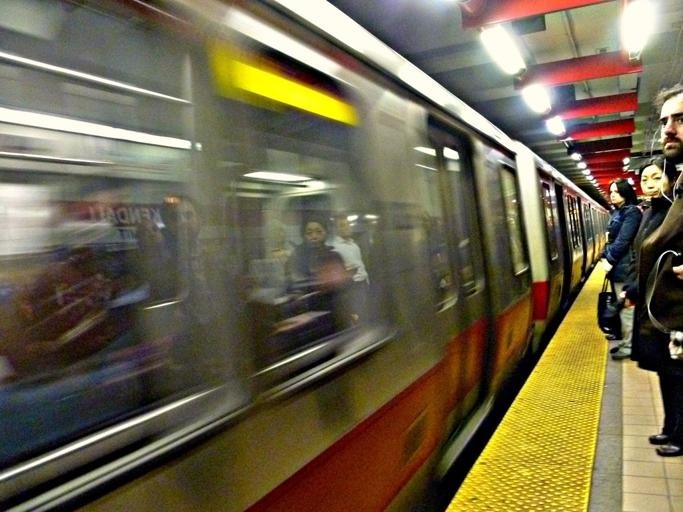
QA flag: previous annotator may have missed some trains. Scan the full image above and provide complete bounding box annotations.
[1,1,616,511]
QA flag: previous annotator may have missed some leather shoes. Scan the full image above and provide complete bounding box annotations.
[649,435,683,456]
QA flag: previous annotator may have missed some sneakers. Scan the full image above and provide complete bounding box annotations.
[610,347,628,360]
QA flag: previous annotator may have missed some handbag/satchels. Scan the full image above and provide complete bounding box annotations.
[597,276,622,340]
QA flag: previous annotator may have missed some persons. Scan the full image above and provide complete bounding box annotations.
[631,84,683,361]
[601,178,647,361]
[1,184,372,459]
[623,152,683,456]
